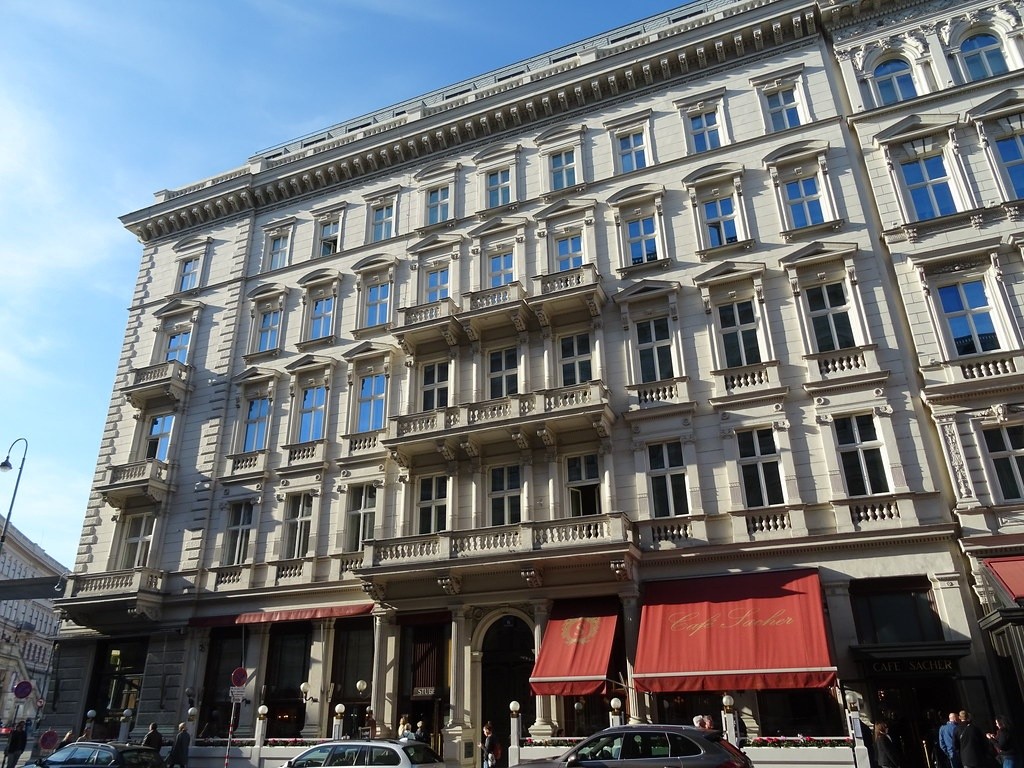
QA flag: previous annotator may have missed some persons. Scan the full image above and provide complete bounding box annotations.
[142,722,163,753]
[363,710,428,745]
[55,727,91,751]
[556,728,565,737]
[874,720,900,768]
[168,721,191,768]
[4,720,27,768]
[477,724,498,768]
[938,709,1020,768]
[692,714,714,731]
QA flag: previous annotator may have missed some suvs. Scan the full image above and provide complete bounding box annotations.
[277,739,446,768]
[18,741,166,768]
[509,724,755,768]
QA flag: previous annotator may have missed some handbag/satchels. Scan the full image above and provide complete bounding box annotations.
[487,753,496,767]
[995,754,1003,764]
[492,743,502,760]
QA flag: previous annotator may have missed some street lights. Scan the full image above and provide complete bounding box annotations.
[0,437,28,552]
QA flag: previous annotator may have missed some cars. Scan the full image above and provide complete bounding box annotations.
[0,719,18,736]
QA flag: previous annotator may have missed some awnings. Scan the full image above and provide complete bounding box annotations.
[633,567,839,692]
[234,602,373,624]
[527,600,621,696]
